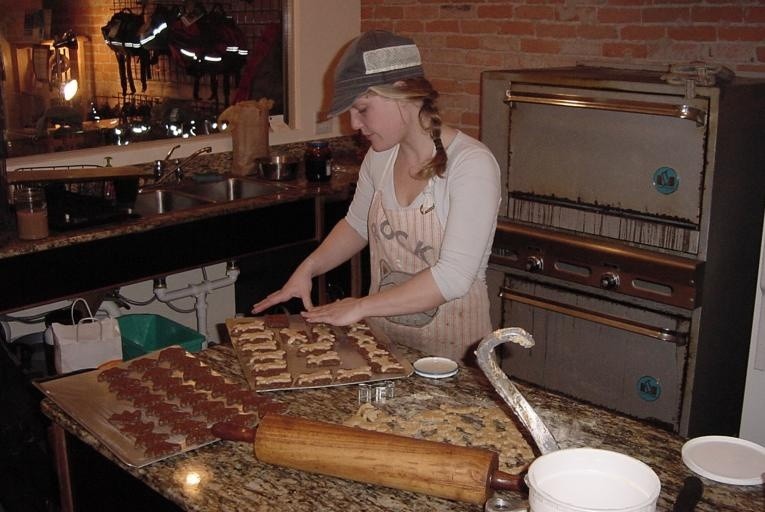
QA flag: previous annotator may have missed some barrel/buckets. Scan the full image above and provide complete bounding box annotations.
[522,447,661,512]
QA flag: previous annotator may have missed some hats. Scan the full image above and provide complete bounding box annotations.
[326,29,425,119]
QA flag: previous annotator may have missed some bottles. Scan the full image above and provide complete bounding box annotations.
[304,139,332,183]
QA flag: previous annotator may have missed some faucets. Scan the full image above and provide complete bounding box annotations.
[155,144,213,186]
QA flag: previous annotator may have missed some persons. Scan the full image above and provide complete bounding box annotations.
[251,30,501,364]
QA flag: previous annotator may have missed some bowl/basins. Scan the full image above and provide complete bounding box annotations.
[254,154,302,181]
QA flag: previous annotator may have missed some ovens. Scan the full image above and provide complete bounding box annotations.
[480,74,708,277]
[482,233,708,436]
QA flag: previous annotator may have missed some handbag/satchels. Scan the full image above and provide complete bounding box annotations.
[51,298,123,375]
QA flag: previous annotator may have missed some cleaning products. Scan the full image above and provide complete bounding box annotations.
[103,156,115,200]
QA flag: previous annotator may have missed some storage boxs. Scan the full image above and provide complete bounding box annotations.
[114,312,205,361]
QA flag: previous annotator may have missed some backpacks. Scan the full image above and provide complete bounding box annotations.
[101,0,246,74]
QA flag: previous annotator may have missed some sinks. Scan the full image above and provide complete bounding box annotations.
[133,186,216,218]
[176,173,297,203]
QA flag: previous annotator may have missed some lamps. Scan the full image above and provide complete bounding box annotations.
[50,35,78,102]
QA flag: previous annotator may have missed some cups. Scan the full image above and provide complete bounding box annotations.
[13,187,48,240]
